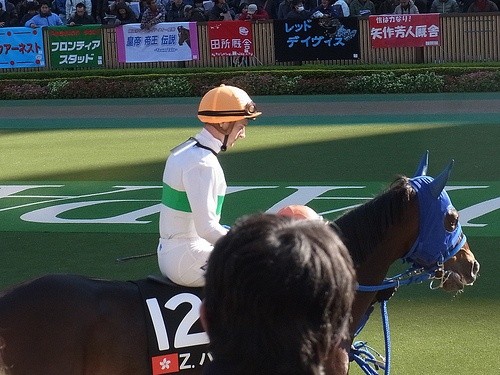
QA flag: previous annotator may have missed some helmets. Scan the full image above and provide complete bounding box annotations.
[197,83,262,125]
[276,205,323,220]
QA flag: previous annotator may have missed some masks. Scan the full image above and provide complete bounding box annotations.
[296,6,304,11]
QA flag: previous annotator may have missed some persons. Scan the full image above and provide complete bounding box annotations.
[157,84,262,287]
[200,213,358,375]
[0,0,500,30]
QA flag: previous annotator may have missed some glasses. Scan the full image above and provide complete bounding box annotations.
[244,100,258,115]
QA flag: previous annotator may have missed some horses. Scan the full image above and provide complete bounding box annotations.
[0,150,480,375]
[177,26,191,49]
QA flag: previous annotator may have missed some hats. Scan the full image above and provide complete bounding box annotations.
[248,4,257,14]
